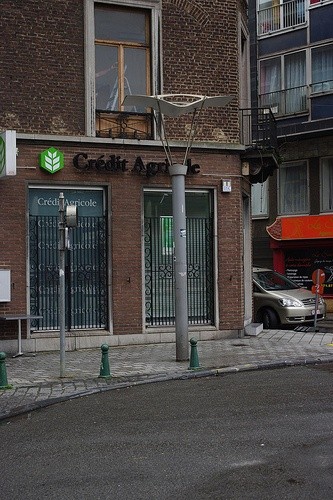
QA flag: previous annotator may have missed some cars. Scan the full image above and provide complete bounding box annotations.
[251,267,325,330]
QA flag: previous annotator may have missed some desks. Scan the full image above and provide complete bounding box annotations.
[0,315,44,357]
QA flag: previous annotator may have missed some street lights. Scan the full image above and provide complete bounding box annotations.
[120,94,235,361]
